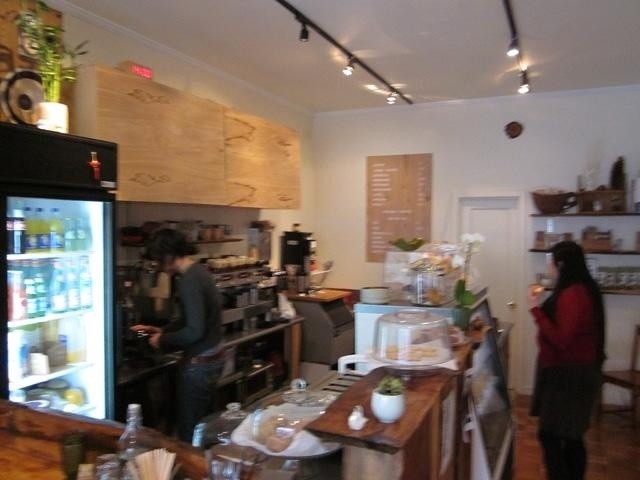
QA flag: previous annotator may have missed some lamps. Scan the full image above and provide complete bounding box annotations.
[497,0,537,98]
[274,0,415,109]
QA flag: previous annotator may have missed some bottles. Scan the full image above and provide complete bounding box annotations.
[88,151,101,181]
[3,203,94,256]
[62,402,156,480]
[4,259,93,321]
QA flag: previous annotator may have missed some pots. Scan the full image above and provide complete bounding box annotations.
[124,221,224,246]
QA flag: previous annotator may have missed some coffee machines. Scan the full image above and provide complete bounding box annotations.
[281,231,317,295]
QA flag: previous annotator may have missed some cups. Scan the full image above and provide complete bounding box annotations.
[532,189,579,213]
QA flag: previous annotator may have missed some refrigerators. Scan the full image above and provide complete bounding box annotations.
[0,121,117,419]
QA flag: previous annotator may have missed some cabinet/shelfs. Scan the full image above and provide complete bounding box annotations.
[58,62,226,210]
[353,285,516,478]
[223,108,305,211]
[526,209,640,297]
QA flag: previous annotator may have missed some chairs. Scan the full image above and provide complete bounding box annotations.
[593,323,639,436]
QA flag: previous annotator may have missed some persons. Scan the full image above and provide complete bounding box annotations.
[129,227,227,437]
[525,241,606,478]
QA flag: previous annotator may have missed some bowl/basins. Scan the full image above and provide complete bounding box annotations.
[360,285,392,305]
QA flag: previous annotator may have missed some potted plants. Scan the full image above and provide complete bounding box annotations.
[368,372,409,426]
[12,2,91,136]
